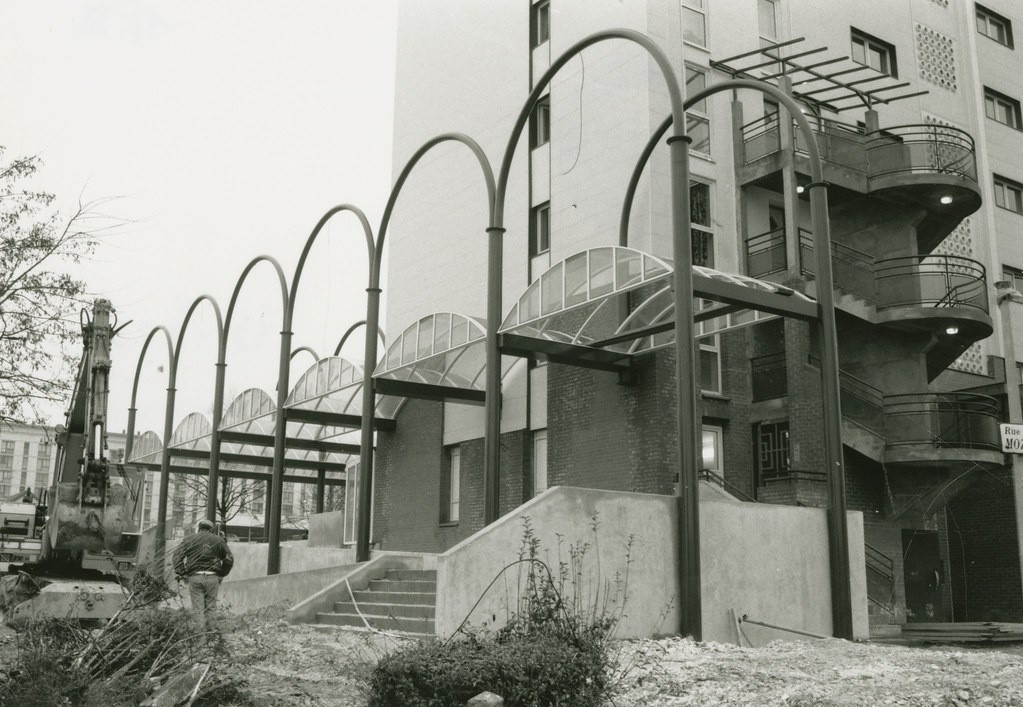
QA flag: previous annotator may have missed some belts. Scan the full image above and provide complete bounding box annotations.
[194,571,217,575]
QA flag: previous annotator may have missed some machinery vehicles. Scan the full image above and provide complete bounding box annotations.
[0,298,156,629]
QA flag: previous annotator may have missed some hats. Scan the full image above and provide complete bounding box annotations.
[198,519,215,529]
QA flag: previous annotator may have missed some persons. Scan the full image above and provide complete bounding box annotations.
[171,519,234,643]
